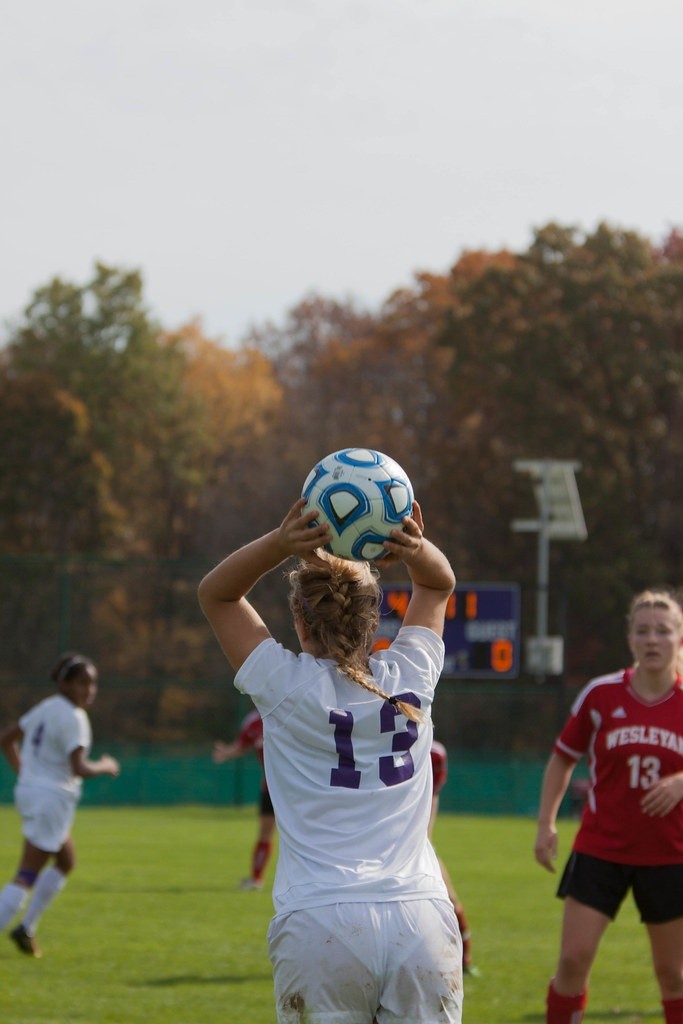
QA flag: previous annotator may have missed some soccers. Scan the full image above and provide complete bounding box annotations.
[299,446,416,564]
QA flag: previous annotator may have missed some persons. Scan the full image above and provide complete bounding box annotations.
[196,496,480,1024]
[0,651,120,959]
[533,589,683,1024]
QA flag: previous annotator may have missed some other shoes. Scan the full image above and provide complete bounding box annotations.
[238,879,262,892]
[12,925,35,954]
